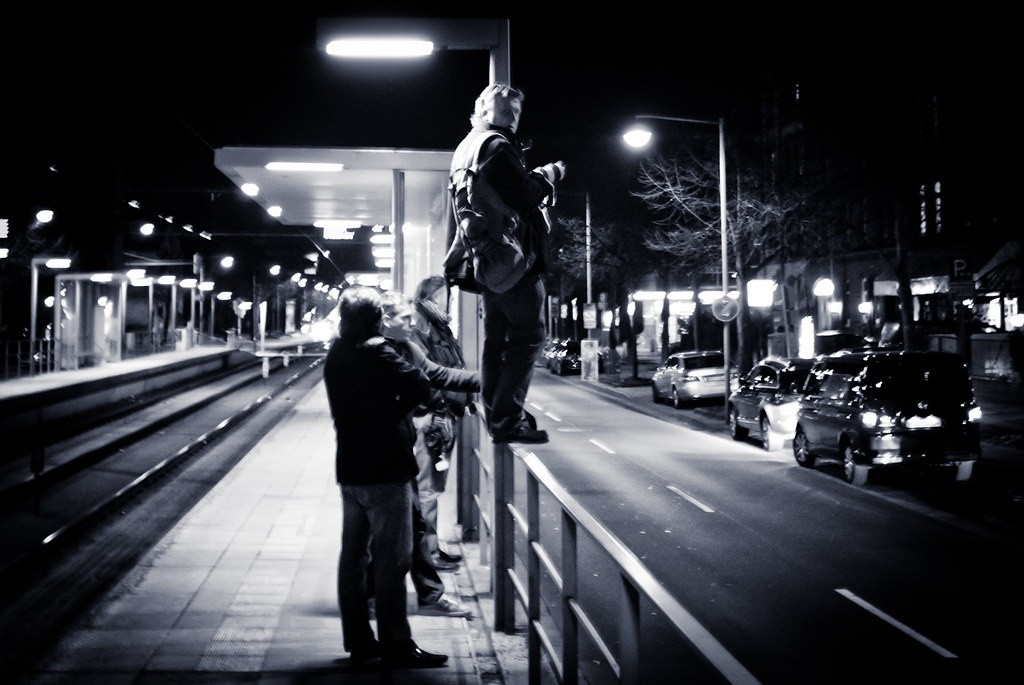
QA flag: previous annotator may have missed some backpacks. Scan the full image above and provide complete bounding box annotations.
[442,166,536,295]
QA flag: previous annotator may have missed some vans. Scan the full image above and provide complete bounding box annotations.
[792,350,983,486]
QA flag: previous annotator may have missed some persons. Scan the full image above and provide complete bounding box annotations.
[323,275,483,671]
[446,80,565,444]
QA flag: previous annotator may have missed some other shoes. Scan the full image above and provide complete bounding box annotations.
[417,595,471,617]
[433,558,460,574]
[492,424,550,445]
[384,641,449,669]
[348,641,382,663]
[369,598,377,620]
[437,549,462,563]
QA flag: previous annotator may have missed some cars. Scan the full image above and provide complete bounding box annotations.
[725,355,818,453]
[538,337,606,376]
[650,350,740,409]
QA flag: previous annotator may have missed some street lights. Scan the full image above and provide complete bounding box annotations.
[620,114,729,434]
[29,257,73,373]
[314,15,511,91]
[55,267,232,370]
